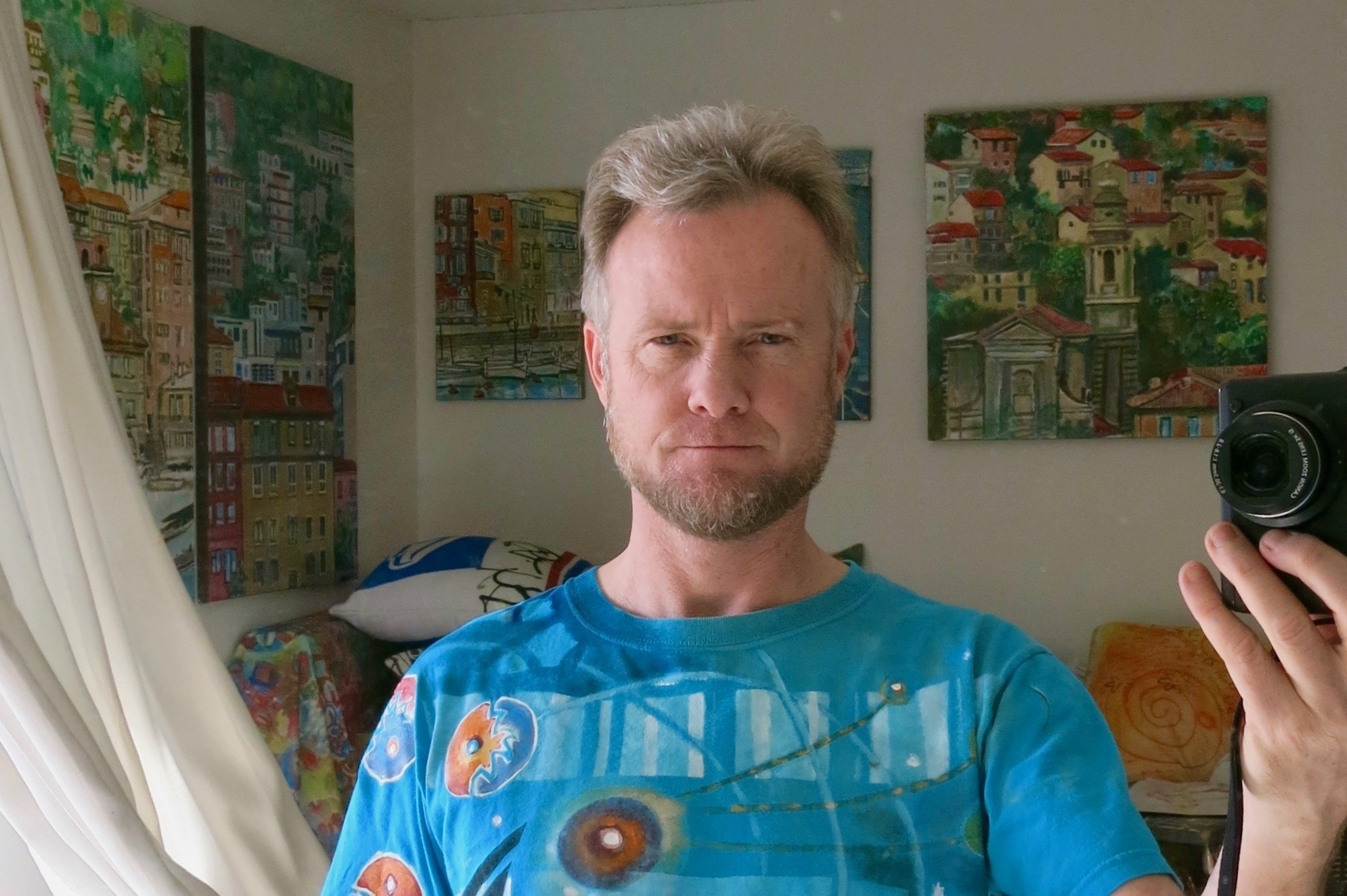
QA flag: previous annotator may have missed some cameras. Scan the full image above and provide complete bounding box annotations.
[1209,368,1347,615]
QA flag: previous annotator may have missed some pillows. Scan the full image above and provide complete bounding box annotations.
[327,533,594,643]
[1083,621,1241,816]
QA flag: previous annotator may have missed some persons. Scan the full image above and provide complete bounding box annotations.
[312,103,1347,896]
